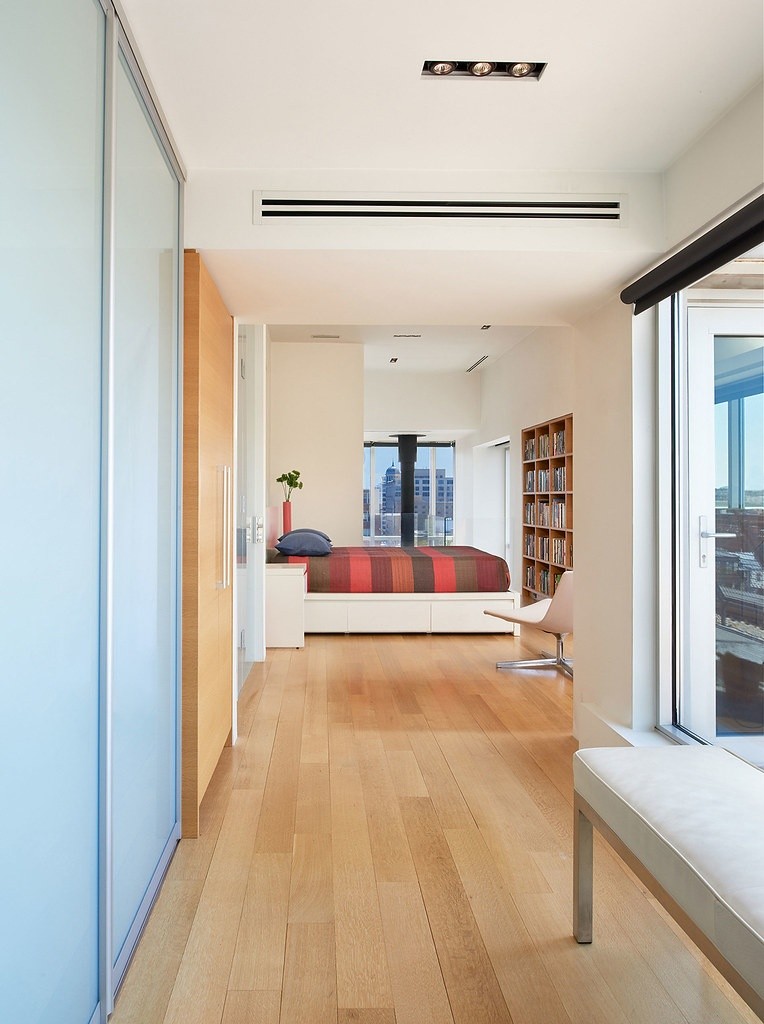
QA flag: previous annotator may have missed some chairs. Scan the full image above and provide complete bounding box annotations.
[483,571,573,678]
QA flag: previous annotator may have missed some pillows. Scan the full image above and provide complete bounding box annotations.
[274,533,334,556]
[276,528,332,543]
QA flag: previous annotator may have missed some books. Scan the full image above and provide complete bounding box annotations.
[525,533,566,566]
[526,467,566,492]
[526,498,565,529]
[526,566,562,595]
[524,430,565,461]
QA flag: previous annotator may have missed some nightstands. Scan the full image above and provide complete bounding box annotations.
[266,563,307,650]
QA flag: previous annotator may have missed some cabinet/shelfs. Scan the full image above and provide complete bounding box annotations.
[521,413,573,601]
[182,249,234,839]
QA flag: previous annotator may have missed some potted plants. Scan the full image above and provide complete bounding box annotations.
[276,469,303,535]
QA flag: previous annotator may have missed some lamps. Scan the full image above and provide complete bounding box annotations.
[506,63,536,78]
[428,61,458,75]
[467,62,497,77]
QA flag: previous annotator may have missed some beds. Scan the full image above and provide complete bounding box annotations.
[266,546,521,637]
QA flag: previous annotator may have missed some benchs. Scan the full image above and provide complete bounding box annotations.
[572,746,764,1024]
